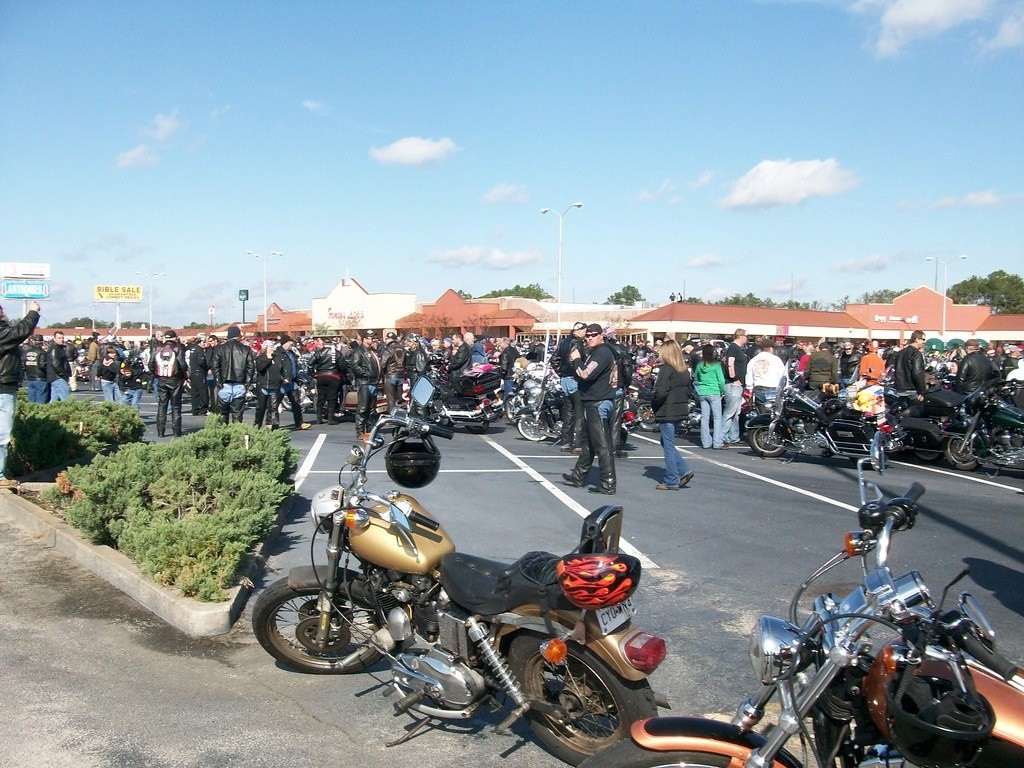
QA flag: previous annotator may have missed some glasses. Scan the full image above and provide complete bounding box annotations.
[585,332,601,338]
[844,347,852,350]
[918,337,925,342]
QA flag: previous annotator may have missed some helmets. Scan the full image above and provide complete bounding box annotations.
[868,341,880,351]
[557,554,642,609]
[886,673,996,768]
[384,441,441,489]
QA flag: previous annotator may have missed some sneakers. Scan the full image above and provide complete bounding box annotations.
[655,484,672,490]
[679,473,694,487]
[296,422,311,429]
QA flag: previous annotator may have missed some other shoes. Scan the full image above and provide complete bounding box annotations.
[572,447,582,453]
[732,440,747,446]
[613,449,628,458]
[560,443,574,452]
[327,420,340,425]
[318,420,324,424]
[358,434,363,439]
[716,444,729,449]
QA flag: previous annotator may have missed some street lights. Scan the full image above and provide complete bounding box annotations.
[248,250,284,332]
[137,271,167,337]
[926,255,968,343]
[541,203,583,346]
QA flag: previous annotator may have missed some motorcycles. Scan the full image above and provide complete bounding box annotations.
[183,357,1024,481]
[575,428,1024,768]
[251,374,672,768]
[393,351,507,435]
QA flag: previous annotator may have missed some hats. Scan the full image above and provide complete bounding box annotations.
[363,329,378,337]
[574,322,587,331]
[585,324,602,335]
[964,339,978,346]
[761,339,774,346]
[280,334,294,345]
[156,331,164,339]
[34,334,44,342]
[228,326,240,338]
[387,332,397,340]
[165,330,177,338]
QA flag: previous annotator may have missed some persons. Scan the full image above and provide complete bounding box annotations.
[0,302,1024,495]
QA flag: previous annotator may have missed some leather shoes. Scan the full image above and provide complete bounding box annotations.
[589,486,615,495]
[562,473,582,487]
